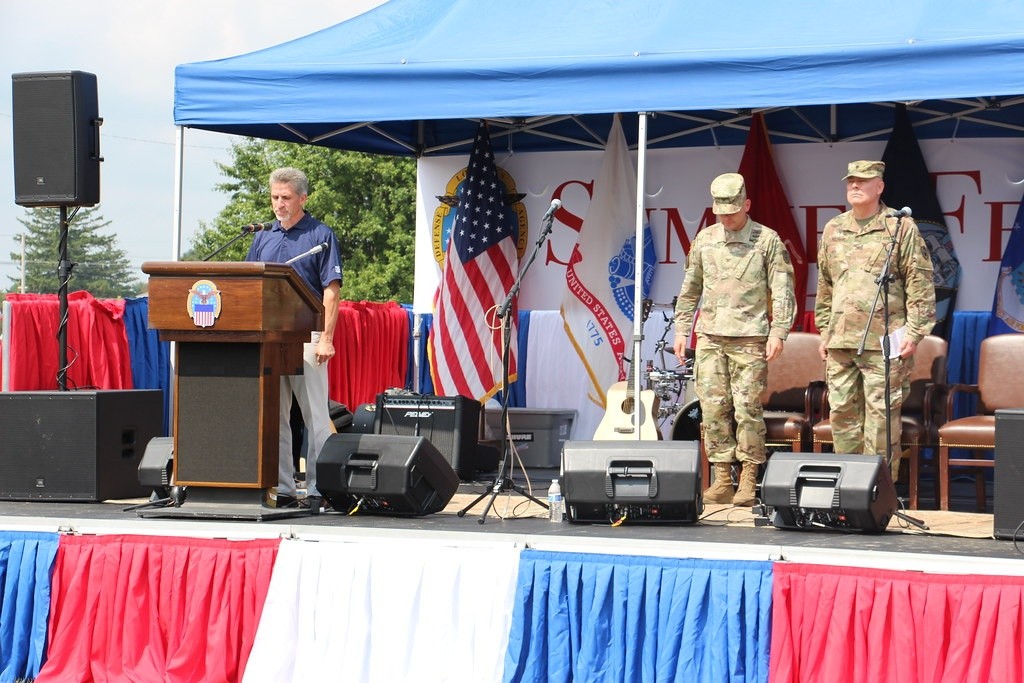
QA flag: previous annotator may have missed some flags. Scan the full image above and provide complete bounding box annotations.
[687,111,807,348]
[985,198,1024,338]
[880,102,961,353]
[425,120,519,406]
[560,113,656,411]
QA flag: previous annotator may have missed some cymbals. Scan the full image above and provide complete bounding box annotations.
[664,347,697,359]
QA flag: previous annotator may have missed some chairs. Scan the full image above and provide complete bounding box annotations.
[937,334,1024,510]
[812,335,949,508]
[697,329,828,503]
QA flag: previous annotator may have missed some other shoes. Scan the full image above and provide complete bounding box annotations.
[300,495,324,508]
[276,496,298,508]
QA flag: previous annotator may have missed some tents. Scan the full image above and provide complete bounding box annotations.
[169,0,1024,438]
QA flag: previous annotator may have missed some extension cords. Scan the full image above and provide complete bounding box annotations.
[754,517,774,526]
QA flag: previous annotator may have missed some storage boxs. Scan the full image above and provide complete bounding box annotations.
[485,407,578,467]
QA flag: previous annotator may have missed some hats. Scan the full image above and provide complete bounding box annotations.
[710,173,746,215]
[841,160,885,182]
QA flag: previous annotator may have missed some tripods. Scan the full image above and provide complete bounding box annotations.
[124,486,186,514]
[858,217,928,532]
[456,216,556,524]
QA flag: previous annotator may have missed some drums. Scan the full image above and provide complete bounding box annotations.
[670,398,705,440]
[685,375,697,403]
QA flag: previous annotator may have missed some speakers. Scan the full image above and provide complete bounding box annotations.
[0,389,165,504]
[561,441,703,526]
[760,452,898,534]
[315,392,485,516]
[994,408,1024,539]
[11,71,101,207]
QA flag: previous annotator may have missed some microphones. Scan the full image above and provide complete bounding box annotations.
[241,222,273,232]
[885,206,912,218]
[542,199,562,220]
[287,242,328,264]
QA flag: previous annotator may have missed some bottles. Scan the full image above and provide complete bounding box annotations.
[548,479,562,523]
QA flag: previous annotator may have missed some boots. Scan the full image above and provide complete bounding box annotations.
[733,461,758,507]
[702,463,735,504]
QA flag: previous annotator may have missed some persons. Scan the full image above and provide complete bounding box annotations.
[673,174,798,506]
[813,158,936,483]
[246,166,342,510]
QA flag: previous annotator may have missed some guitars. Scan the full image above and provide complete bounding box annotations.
[592,298,665,441]
